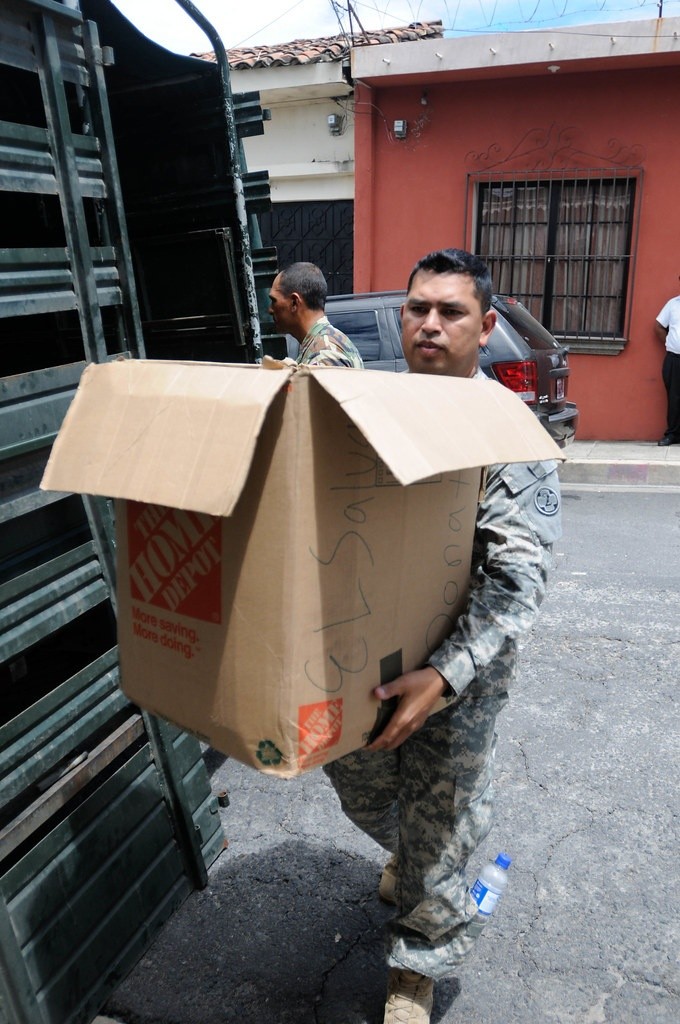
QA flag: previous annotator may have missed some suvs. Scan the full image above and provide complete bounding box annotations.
[268,288,579,449]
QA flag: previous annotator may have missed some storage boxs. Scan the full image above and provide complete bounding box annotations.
[40,359,567,783]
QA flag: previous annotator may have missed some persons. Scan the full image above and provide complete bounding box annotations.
[268,261,365,369]
[320,250,562,1024]
[654,276,680,446]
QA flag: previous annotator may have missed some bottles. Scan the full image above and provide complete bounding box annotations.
[468,854,512,935]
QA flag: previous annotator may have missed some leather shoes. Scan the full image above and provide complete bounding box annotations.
[658,435,680,446]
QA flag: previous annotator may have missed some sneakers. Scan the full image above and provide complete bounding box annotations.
[378,854,398,902]
[384,967,434,1024]
